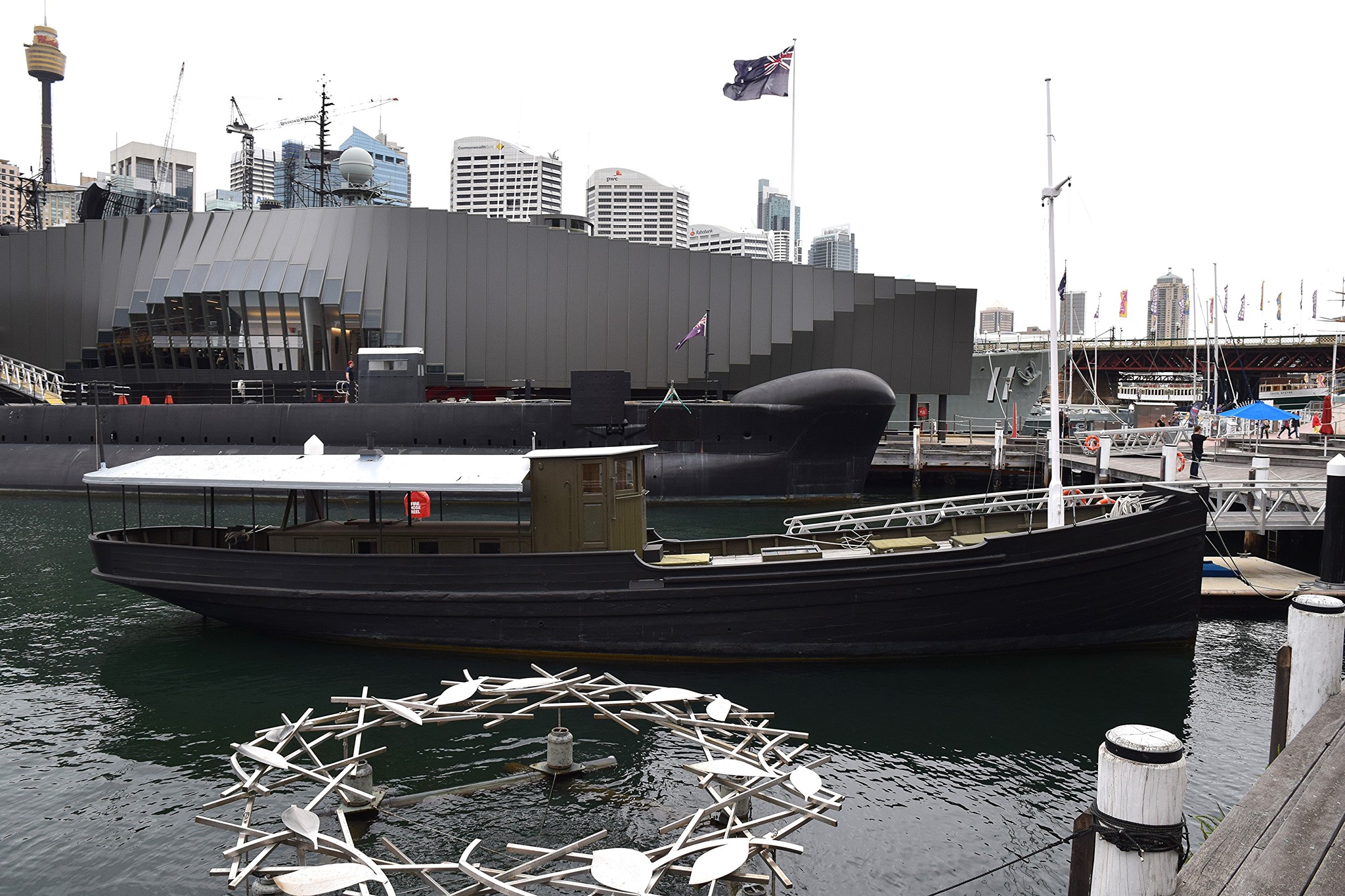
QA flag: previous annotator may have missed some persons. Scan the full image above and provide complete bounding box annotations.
[1216,404,1222,419]
[1155,411,1180,443]
[1059,411,1071,439]
[344,360,356,403]
[1312,412,1321,434]
[1277,411,1300,438]
[216,348,229,369]
[1259,419,1269,439]
[1189,426,1224,479]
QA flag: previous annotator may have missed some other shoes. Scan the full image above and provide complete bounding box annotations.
[1189,476,1192,478]
[1296,436,1299,438]
[1193,476,1202,479]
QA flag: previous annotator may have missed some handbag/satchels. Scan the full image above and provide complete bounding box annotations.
[1070,423,1073,428]
[1284,421,1289,427]
[1066,421,1071,427]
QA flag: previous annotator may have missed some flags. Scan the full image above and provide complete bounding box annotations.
[1058,267,1066,300]
[1094,277,1345,324]
[675,311,707,352]
[723,45,794,101]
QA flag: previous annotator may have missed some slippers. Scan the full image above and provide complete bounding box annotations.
[1288,436,1292,438]
[1276,436,1280,439]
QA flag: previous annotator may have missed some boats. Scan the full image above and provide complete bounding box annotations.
[882,334,1072,435]
[1258,387,1330,414]
[82,75,1212,664]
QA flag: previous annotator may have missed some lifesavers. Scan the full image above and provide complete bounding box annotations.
[1085,435,1099,451]
[1095,497,1116,505]
[1063,489,1087,506]
[1176,451,1185,472]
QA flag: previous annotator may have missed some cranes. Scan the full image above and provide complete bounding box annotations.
[149,62,186,214]
[225,95,399,210]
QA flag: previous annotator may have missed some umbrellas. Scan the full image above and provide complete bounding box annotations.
[1318,395,1334,456]
[1217,400,1302,455]
